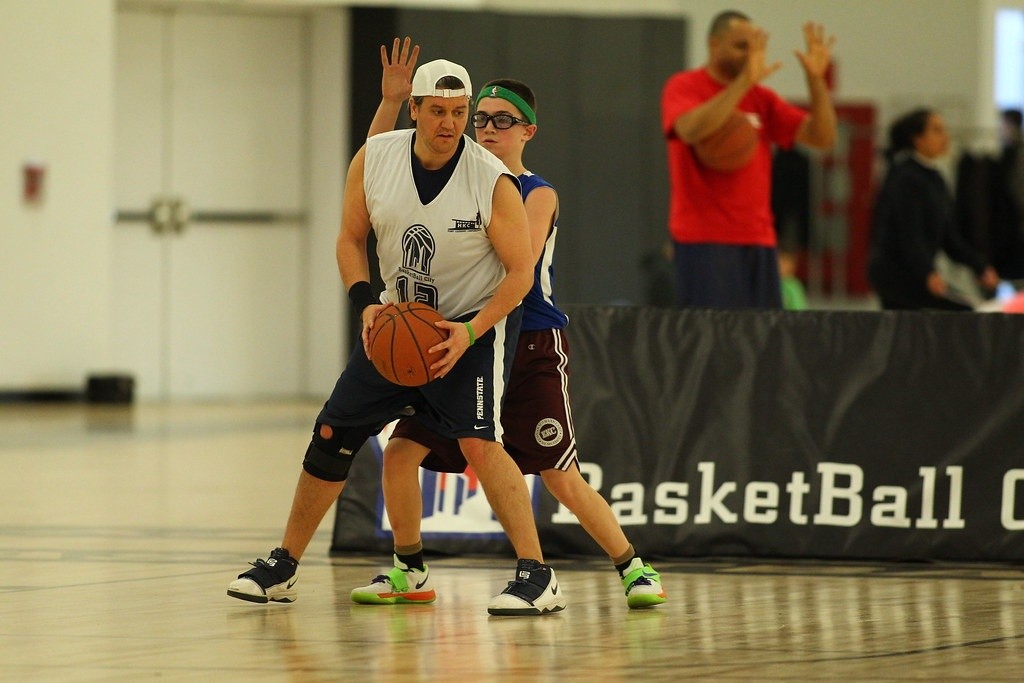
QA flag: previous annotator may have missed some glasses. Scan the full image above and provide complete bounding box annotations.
[471,113,529,129]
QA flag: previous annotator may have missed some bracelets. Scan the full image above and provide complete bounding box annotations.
[349,281,378,317]
[465,323,474,345]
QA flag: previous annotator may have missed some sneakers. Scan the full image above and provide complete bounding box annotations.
[227,547,300,603]
[487,558,565,615]
[622,562,665,609]
[350,554,436,605]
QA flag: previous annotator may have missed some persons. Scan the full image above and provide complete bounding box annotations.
[661,11,839,308]
[870,108,1024,310]
[227,59,567,615]
[351,37,665,608]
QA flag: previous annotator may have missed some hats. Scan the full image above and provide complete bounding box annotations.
[411,58,473,98]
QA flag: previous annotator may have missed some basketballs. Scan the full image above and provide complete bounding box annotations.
[369,302,450,388]
